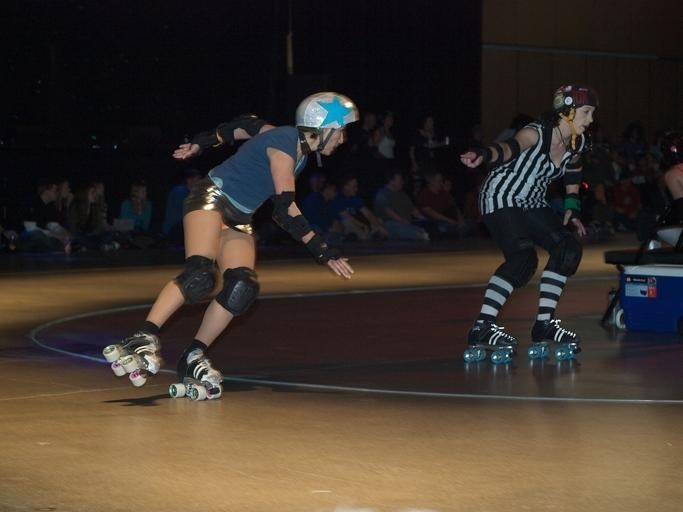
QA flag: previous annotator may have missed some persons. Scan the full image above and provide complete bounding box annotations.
[122,92,360,388]
[296,114,490,240]
[459,84,600,350]
[2,168,202,253]
[568,125,683,248]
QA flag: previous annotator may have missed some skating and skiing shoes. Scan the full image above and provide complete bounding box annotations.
[103,331,161,387]
[464,320,518,364]
[169,348,223,401]
[528,320,581,359]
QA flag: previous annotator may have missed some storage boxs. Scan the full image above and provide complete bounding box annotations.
[619,264,683,334]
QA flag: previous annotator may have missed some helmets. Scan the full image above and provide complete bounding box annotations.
[295,92,360,129]
[553,84,598,109]
